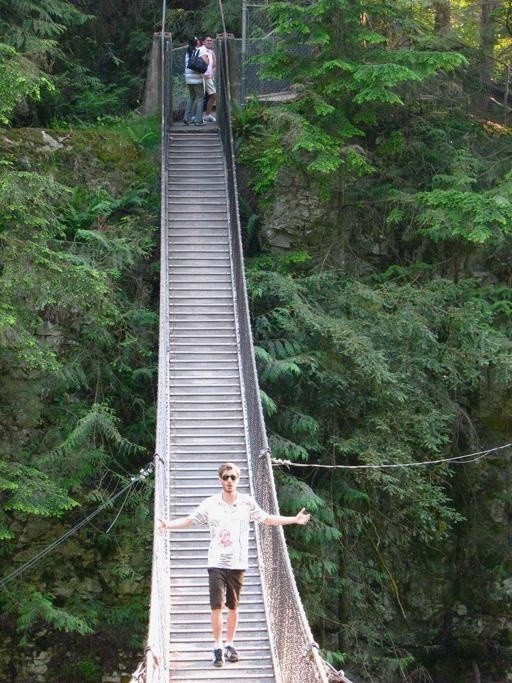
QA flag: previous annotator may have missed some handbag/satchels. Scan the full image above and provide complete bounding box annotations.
[187,57,207,73]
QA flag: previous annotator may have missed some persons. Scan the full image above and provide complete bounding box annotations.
[181,35,208,126]
[199,34,218,122]
[155,460,313,668]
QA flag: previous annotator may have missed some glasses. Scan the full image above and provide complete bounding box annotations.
[222,474,237,480]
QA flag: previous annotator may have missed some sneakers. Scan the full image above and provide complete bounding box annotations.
[224,646,239,662]
[183,115,216,126]
[213,649,224,667]
